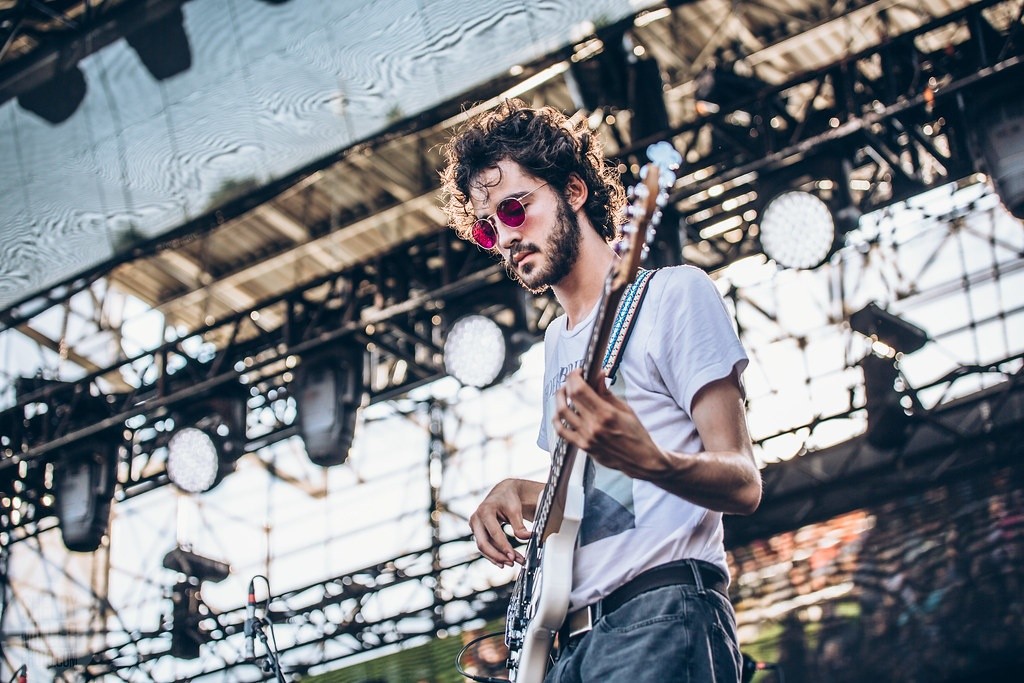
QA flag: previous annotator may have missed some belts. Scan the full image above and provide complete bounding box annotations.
[558,565,730,647]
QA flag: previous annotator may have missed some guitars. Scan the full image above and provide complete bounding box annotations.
[503,140,683,683]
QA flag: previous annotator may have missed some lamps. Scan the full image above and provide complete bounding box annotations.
[754,177,861,273]
[165,423,239,495]
[440,313,535,392]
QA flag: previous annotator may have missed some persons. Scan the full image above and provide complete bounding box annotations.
[441,107,762,683]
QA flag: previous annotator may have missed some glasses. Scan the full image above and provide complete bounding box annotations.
[471,182,547,249]
